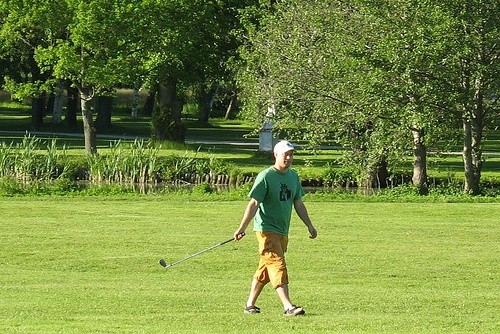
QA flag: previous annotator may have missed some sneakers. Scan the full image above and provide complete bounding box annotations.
[285,305,305,315]
[244,304,260,315]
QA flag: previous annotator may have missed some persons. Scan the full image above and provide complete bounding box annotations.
[234,140,317,315]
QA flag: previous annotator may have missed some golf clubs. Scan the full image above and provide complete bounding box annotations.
[157,232,246,271]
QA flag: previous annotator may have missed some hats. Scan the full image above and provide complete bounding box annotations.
[274,140,294,153]
